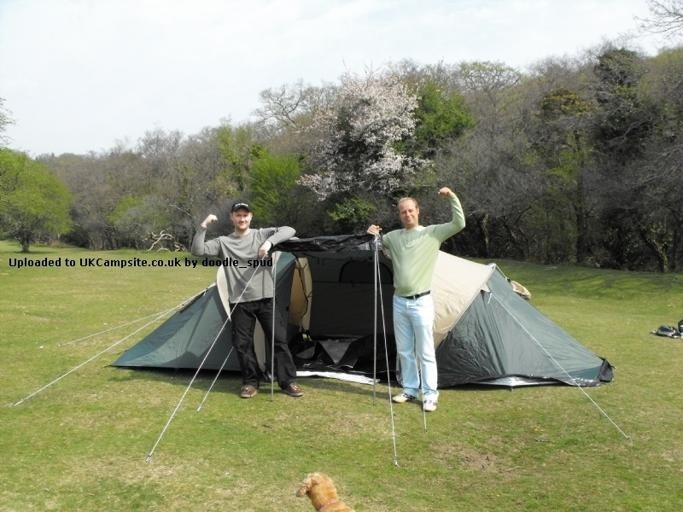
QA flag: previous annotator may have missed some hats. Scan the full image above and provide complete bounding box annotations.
[232,202,253,212]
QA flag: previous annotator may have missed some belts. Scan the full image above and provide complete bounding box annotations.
[403,291,431,300]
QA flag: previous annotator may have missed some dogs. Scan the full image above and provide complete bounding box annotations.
[296,472,353,512]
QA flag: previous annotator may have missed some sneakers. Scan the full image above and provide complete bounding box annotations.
[392,391,415,403]
[284,383,306,398]
[422,400,437,413]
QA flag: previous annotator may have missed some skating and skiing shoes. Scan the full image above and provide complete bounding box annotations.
[239,384,258,400]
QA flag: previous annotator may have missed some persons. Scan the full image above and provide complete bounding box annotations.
[369,186,466,411]
[192,199,305,397]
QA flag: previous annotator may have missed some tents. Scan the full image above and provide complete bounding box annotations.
[105,234,613,388]
